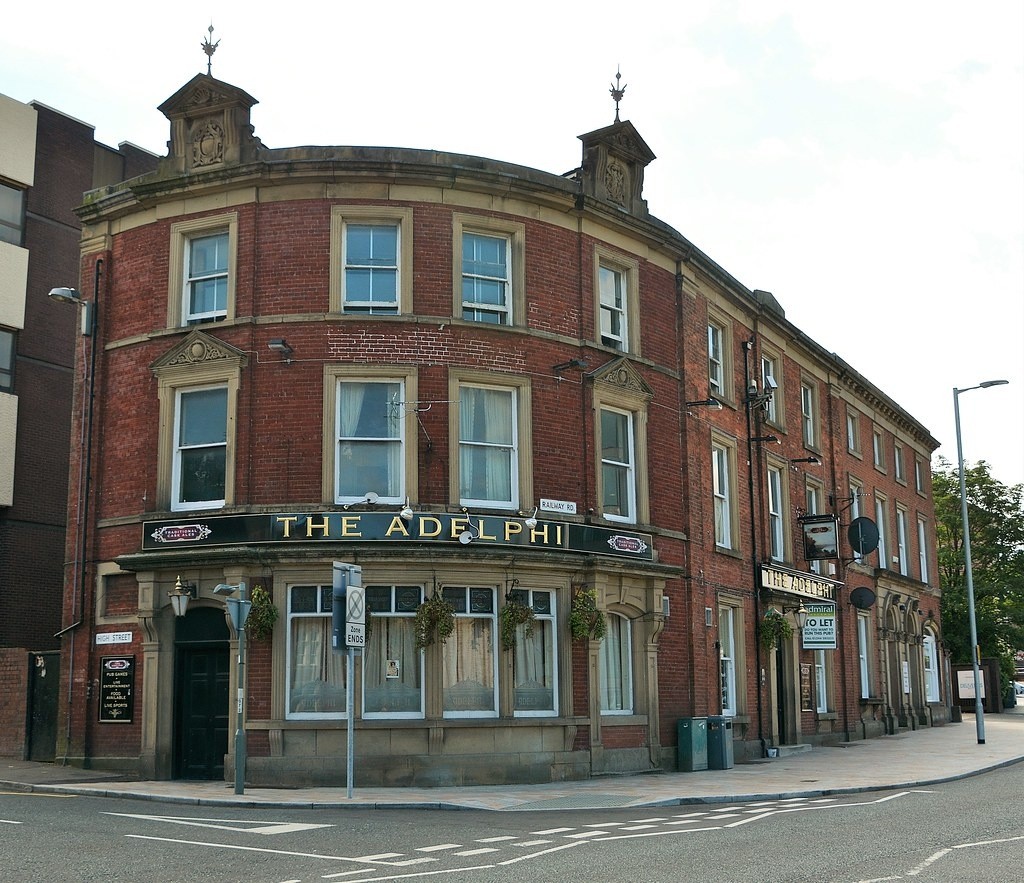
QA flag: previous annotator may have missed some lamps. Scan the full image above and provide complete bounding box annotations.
[168,574,197,620]
[459,507,480,545]
[751,435,782,445]
[686,398,722,411]
[518,506,537,530]
[344,492,379,510]
[268,339,293,360]
[400,497,414,520]
[792,457,822,467]
[553,358,588,372]
[48,287,92,337]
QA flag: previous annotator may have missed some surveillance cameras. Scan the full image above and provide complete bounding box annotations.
[765,376,778,390]
[748,380,758,393]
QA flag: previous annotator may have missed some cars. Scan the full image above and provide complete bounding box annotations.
[1013,681,1024,695]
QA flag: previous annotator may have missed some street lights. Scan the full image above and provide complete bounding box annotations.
[212,582,245,794]
[952,379,1009,746]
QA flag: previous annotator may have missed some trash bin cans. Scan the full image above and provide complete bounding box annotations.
[677,716,708,771]
[708,714,735,770]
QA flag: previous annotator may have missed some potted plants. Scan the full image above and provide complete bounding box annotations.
[497,592,537,651]
[568,590,607,643]
[412,594,458,654]
[757,608,792,652]
[782,599,808,638]
